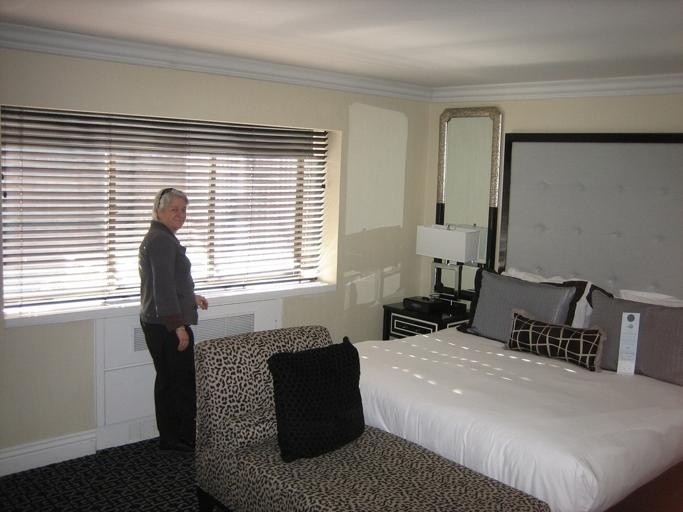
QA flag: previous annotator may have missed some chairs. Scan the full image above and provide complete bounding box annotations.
[194,322,547,511]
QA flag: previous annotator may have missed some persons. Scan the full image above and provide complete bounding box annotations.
[135,186,210,452]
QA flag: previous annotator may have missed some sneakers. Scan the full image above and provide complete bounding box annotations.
[168,438,195,451]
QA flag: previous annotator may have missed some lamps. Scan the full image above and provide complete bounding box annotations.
[455,222,489,267]
[416,224,479,318]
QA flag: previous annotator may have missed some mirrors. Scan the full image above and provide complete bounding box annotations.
[432,104,503,304]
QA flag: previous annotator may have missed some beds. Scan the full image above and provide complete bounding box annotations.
[340,325,683,512]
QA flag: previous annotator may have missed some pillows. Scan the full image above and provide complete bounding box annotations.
[465,269,683,389]
[266,335,367,465]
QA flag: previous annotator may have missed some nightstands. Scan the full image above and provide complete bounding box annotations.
[381,295,471,342]
